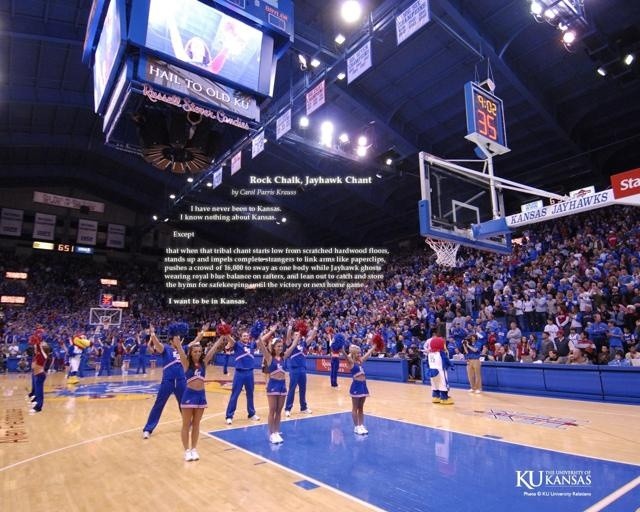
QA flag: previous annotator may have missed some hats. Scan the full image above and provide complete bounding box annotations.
[470,334,478,338]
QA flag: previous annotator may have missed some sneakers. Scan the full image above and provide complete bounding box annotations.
[469,389,474,392]
[285,411,291,417]
[354,426,364,434]
[331,384,338,386]
[275,432,284,442]
[270,433,279,443]
[29,409,42,412]
[248,415,260,421]
[184,449,193,461]
[190,448,199,460]
[144,431,150,439]
[475,389,481,394]
[226,419,232,425]
[25,393,37,407]
[301,408,312,414]
[360,424,368,433]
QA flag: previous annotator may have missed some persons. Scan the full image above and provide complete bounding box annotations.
[166,9,235,75]
[0,205,640,462]
